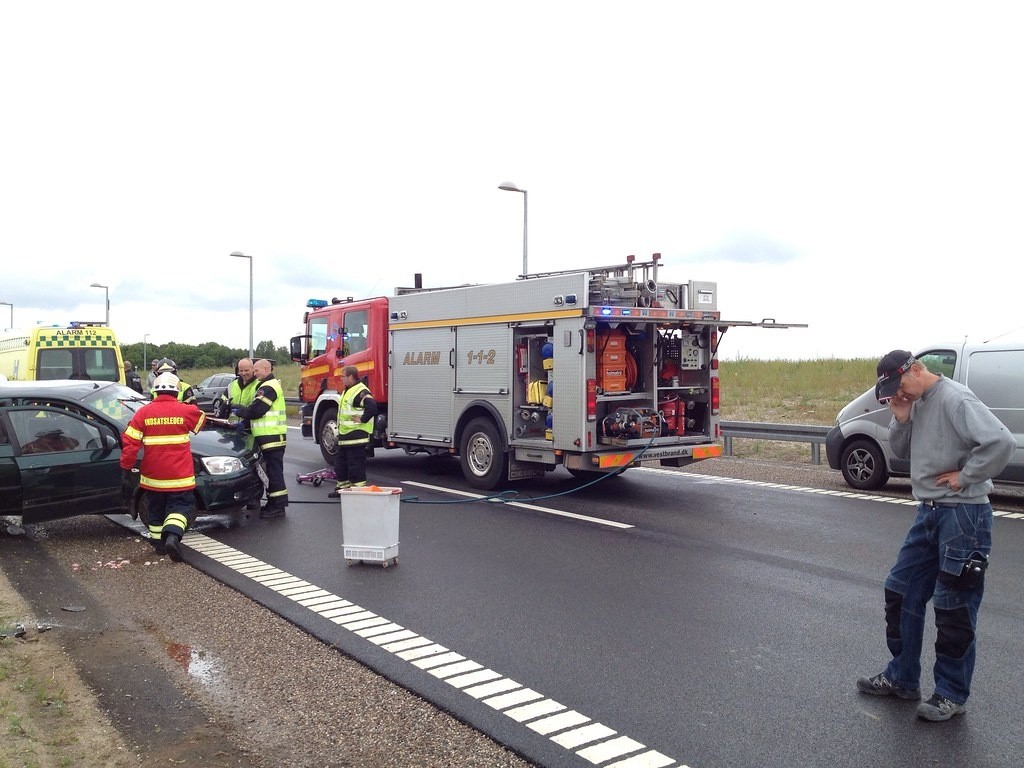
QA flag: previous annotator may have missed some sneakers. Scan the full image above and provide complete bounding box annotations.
[855,670,920,699]
[916,690,966,721]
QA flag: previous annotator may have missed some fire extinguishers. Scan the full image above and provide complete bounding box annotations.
[661,392,685,436]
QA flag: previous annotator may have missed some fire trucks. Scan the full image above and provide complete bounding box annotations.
[290,254,809,492]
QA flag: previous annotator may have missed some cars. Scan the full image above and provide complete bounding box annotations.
[0,380,269,526]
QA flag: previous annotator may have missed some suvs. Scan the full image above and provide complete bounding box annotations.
[191,373,239,418]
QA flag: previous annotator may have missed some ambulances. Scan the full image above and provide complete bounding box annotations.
[0,322,127,420]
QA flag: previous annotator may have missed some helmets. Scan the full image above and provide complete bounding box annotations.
[153,371,182,392]
[156,358,178,378]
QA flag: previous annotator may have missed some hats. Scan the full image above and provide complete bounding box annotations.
[877,350,915,402]
[151,358,159,365]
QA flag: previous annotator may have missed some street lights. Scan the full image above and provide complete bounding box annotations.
[0,302,13,329]
[144,333,151,371]
[229,250,253,361]
[498,180,527,277]
[90,283,109,327]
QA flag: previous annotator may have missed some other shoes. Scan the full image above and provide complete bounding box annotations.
[328,487,341,497]
[261,502,270,510]
[155,544,167,555]
[261,506,286,518]
[165,533,184,561]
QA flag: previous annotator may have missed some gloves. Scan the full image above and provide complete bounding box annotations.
[231,408,237,415]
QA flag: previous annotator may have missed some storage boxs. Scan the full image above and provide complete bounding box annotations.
[595,334,626,393]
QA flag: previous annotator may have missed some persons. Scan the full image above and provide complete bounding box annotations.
[856,350,1017,721]
[148,356,197,406]
[328,366,378,499]
[124,361,143,394]
[218,357,261,508]
[22,427,79,454]
[222,359,289,517]
[120,372,206,562]
[146,359,159,388]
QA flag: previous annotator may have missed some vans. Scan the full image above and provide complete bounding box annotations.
[825,340,1024,490]
